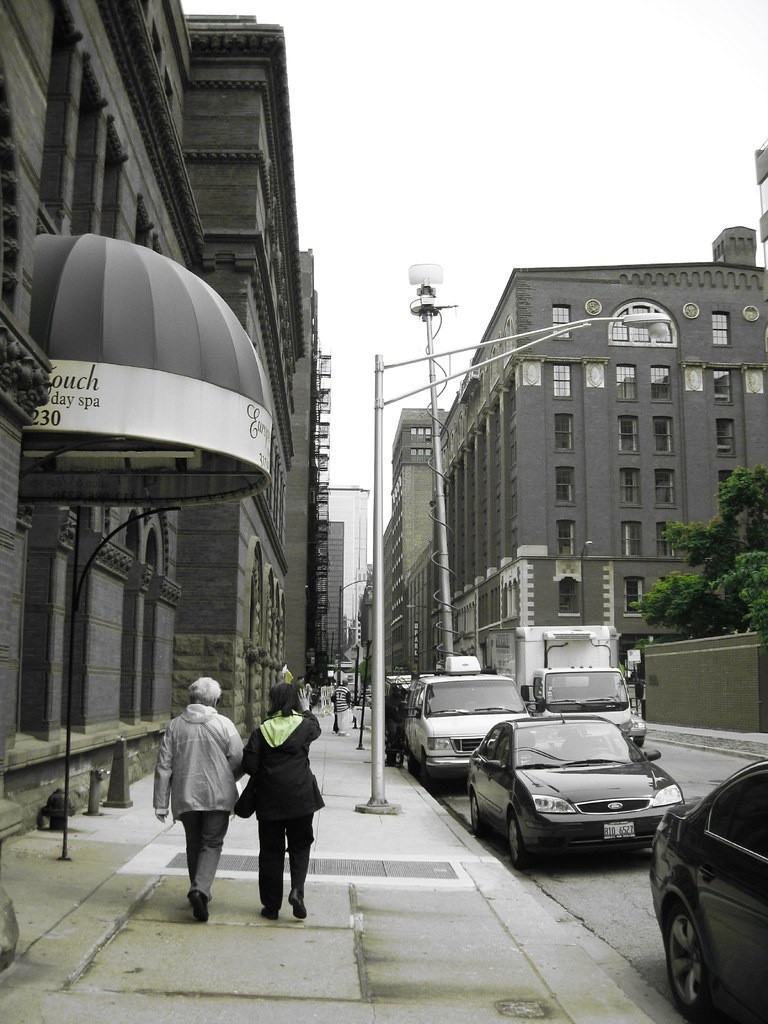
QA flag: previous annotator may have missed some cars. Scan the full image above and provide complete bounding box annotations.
[464,715,684,871]
[627,708,647,747]
[648,762,768,1022]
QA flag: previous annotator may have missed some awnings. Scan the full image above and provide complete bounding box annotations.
[23,235,272,505]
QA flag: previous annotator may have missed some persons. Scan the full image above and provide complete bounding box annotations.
[385,685,406,764]
[331,679,352,736]
[241,683,326,920]
[154,678,244,921]
[292,676,305,696]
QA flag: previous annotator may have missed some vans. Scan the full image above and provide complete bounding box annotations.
[402,655,530,784]
[384,674,437,711]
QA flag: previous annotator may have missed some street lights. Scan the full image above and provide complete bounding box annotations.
[354,313,674,819]
[410,263,459,659]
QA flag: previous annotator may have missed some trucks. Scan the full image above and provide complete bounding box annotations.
[481,625,645,740]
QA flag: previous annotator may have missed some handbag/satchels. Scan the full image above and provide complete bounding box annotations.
[233,777,262,818]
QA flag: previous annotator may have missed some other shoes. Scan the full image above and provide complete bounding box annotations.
[189,890,208,921]
[289,889,307,919]
[261,907,278,920]
